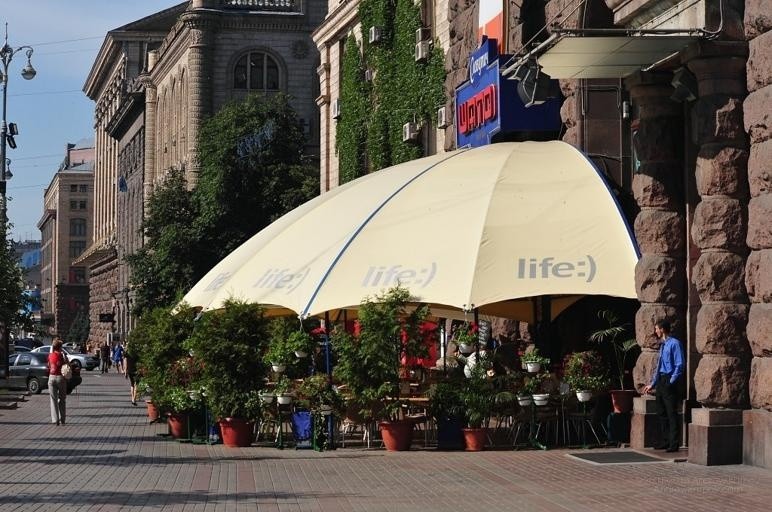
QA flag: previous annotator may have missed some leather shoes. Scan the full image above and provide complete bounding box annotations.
[654,443,680,453]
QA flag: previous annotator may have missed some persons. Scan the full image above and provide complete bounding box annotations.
[112,342,127,374]
[99,342,111,374]
[45,338,73,425]
[642,320,684,451]
[122,350,142,407]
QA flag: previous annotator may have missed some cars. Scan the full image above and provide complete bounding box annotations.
[7,351,83,397]
[7,333,100,372]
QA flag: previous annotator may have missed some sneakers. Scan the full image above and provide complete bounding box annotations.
[55,418,68,426]
[131,400,137,407]
[99,367,128,380]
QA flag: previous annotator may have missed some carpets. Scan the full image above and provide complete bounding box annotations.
[569,451,673,465]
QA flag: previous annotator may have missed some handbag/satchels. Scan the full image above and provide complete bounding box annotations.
[60,351,74,381]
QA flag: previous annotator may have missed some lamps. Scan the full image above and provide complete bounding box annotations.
[507,59,550,108]
[670,66,698,104]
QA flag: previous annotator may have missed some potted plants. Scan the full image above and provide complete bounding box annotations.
[560,349,613,403]
[586,308,638,415]
[328,280,431,450]
[423,343,520,452]
[520,348,552,374]
[448,308,480,355]
[514,370,551,407]
[531,392,551,406]
[127,288,337,447]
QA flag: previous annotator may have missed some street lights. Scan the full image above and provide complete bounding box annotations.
[3,155,16,237]
[0,17,39,380]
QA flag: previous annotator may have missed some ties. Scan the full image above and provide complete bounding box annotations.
[657,344,665,377]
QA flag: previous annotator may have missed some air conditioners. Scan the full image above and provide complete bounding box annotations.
[403,122,422,143]
[416,28,432,44]
[369,26,381,44]
[330,98,341,119]
[415,40,433,63]
[437,107,448,128]
[365,69,372,82]
